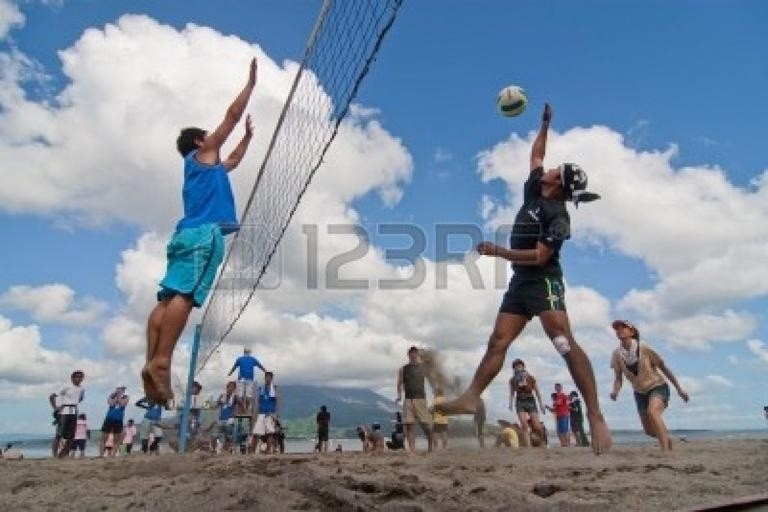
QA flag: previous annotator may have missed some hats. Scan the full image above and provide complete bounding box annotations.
[612,319,635,333]
[116,384,126,389]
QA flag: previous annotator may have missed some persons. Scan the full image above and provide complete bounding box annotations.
[608,318,690,452]
[313,358,591,455]
[44,346,290,458]
[395,344,435,453]
[430,102,614,457]
[142,55,259,412]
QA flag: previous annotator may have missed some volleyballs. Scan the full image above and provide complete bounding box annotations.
[497,86,528,117]
